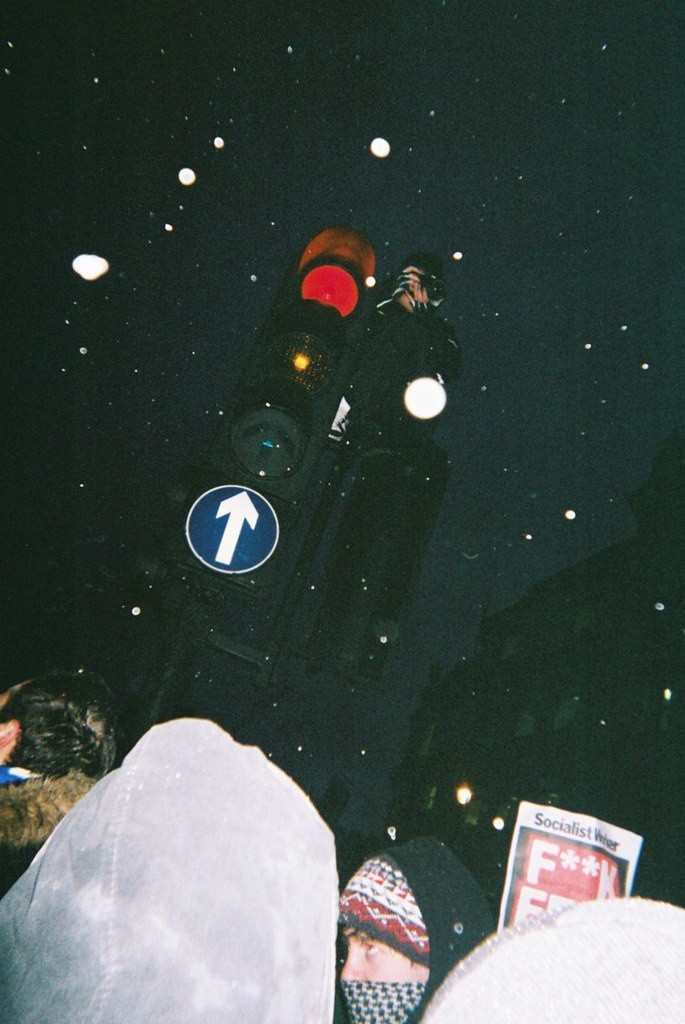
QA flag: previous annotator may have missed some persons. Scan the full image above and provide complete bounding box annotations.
[0,672,685,1024]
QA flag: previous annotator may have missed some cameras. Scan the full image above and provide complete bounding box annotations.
[408,271,446,303]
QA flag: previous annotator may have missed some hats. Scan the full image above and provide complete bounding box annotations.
[338,852,430,968]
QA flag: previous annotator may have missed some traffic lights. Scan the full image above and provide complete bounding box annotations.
[225,221,377,500]
